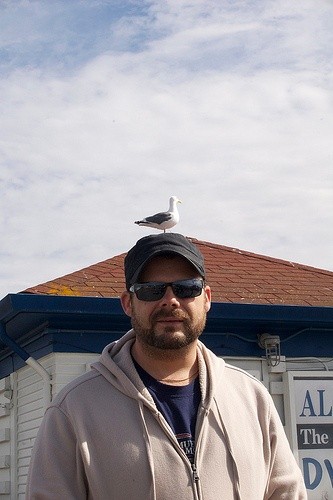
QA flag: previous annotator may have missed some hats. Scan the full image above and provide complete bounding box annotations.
[123,233,206,290]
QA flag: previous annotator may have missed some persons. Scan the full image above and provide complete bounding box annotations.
[25,233,309,500]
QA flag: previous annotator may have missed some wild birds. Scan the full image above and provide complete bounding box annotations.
[134,196,183,232]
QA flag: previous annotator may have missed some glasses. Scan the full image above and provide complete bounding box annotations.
[129,279,207,301]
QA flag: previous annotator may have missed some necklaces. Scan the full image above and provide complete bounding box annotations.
[155,370,199,382]
